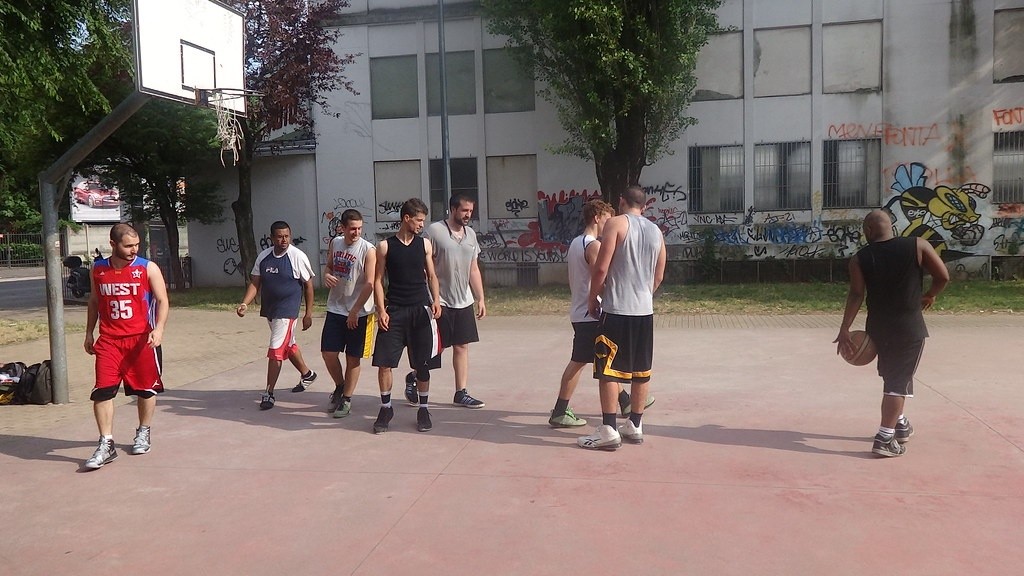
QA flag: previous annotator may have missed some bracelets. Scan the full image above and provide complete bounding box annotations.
[241,303,247,310]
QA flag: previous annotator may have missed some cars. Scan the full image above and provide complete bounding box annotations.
[74,180,121,209]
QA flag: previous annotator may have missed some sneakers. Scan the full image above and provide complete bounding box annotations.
[260,385,275,409]
[374,406,394,432]
[417,407,433,431]
[86,435,118,468]
[454,388,485,408]
[549,407,587,426]
[292,370,317,392]
[620,394,655,416]
[872,434,906,456]
[404,372,418,403]
[132,425,151,453]
[618,418,644,443]
[328,393,341,411]
[577,424,621,448]
[894,417,913,442]
[333,398,352,417]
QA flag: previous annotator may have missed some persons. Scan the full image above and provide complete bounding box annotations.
[85,223,170,468]
[371,198,441,434]
[831,210,951,455]
[549,198,656,429]
[320,208,378,417]
[403,190,487,408]
[577,187,668,448]
[237,221,317,408]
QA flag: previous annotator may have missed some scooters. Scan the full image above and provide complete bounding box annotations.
[63,248,100,298]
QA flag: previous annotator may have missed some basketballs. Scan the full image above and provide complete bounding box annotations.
[839,330,878,366]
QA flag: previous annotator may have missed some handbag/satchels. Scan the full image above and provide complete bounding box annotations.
[0,360,51,405]
